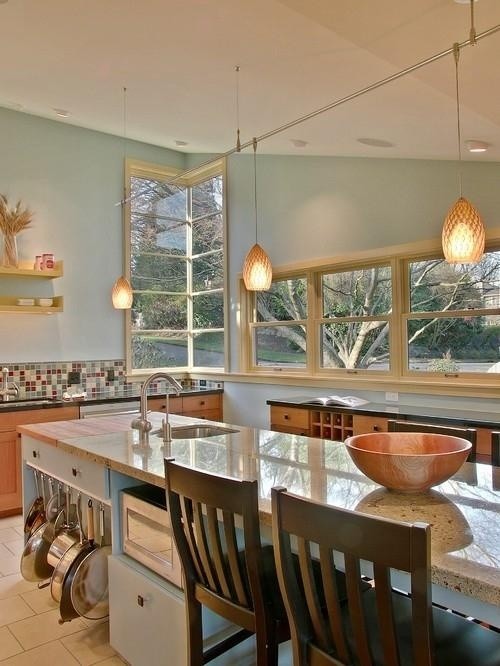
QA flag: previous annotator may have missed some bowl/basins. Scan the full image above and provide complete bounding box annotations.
[342,431,472,493]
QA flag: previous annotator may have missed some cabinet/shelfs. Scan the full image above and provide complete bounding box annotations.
[182,394,222,422]
[270,404,310,438]
[1,259,65,314]
[145,398,184,414]
[107,552,235,666]
[308,410,353,443]
[351,414,394,437]
[21,434,147,554]
[0,406,80,512]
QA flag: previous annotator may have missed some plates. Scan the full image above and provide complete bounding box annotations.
[17,298,53,307]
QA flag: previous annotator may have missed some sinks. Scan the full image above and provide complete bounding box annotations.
[153,424,242,439]
[0,398,63,408]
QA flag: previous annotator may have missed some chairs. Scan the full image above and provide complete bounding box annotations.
[162,418,500,665]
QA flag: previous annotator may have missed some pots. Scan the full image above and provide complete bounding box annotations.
[20,468,112,625]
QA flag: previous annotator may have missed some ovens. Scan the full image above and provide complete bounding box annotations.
[78,401,141,419]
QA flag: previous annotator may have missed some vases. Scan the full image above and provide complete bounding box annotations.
[4,234,19,267]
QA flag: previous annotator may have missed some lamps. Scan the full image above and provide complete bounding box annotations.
[112,202,134,310]
[441,42,484,266]
[243,134,276,293]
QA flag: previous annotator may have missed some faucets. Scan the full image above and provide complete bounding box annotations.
[0,367,19,401]
[131,368,182,442]
[162,386,180,443]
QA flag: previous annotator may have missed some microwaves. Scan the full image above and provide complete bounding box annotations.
[121,482,206,593]
